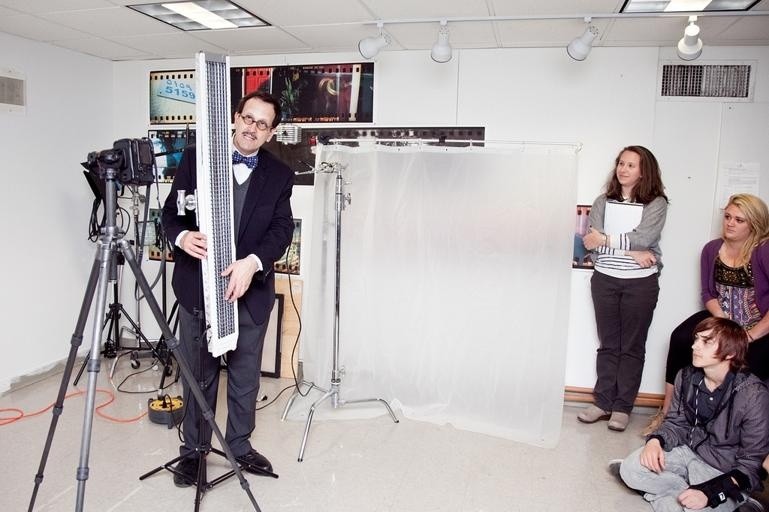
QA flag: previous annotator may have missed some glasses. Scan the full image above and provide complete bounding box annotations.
[240,113,274,131]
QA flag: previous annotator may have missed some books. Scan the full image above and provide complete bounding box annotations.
[604,200,644,236]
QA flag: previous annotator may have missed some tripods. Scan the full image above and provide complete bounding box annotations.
[156,299,181,389]
[282,171,397,461]
[26,167,264,512]
[73,278,166,387]
[109,202,172,376]
[140,255,262,512]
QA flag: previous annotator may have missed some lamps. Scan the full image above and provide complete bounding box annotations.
[356,34,392,59]
[430,25,451,61]
[566,27,597,62]
[677,25,702,60]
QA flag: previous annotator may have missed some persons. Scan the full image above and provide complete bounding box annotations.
[640,193,769,438]
[608,316,769,512]
[573,146,669,432]
[155,91,293,488]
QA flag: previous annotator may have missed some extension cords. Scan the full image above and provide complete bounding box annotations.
[147,395,186,424]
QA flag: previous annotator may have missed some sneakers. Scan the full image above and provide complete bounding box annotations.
[609,458,624,478]
[640,405,665,436]
[739,497,765,511]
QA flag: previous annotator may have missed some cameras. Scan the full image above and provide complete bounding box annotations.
[114,136,155,186]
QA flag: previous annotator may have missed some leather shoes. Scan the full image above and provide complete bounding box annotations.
[174,456,199,488]
[577,404,611,423]
[608,411,630,431]
[235,448,274,475]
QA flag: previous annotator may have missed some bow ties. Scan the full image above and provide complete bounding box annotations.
[232,150,259,170]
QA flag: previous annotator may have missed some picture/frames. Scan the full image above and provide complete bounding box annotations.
[218,294,283,379]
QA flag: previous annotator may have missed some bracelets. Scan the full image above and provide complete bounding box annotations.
[605,235,607,247]
[747,330,754,342]
